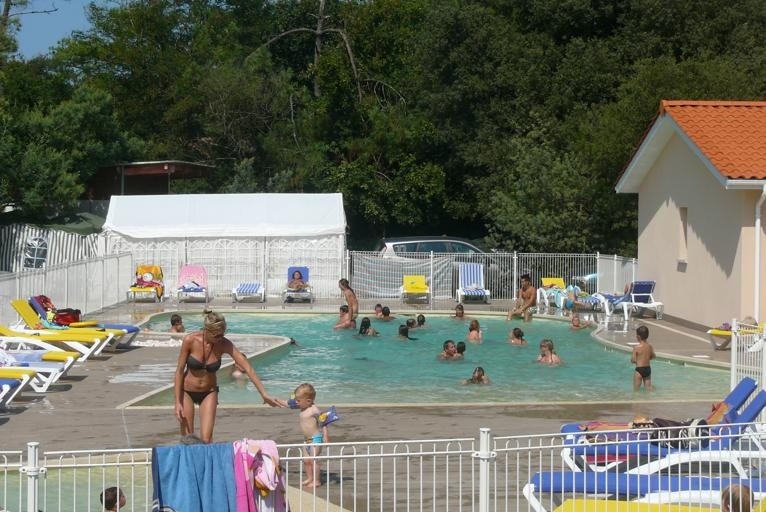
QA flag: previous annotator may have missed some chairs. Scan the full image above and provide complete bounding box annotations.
[706,323,766,350]
[537,278,664,321]
[282,266,313,309]
[523,376,766,512]
[0,296,141,406]
[232,283,265,302]
[456,263,490,304]
[126,264,163,303]
[170,264,208,305]
[399,275,430,305]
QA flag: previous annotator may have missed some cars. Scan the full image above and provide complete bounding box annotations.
[570,265,597,294]
[371,236,532,299]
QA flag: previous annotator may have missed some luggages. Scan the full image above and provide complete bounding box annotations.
[28,293,82,326]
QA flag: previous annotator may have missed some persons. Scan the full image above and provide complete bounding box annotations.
[536,338,563,367]
[459,366,493,386]
[721,483,754,512]
[168,315,186,334]
[231,352,250,381]
[173,307,285,444]
[331,278,483,365]
[507,327,530,346]
[99,487,126,512]
[287,270,309,290]
[630,325,656,393]
[505,274,537,325]
[569,315,590,333]
[276,383,327,488]
